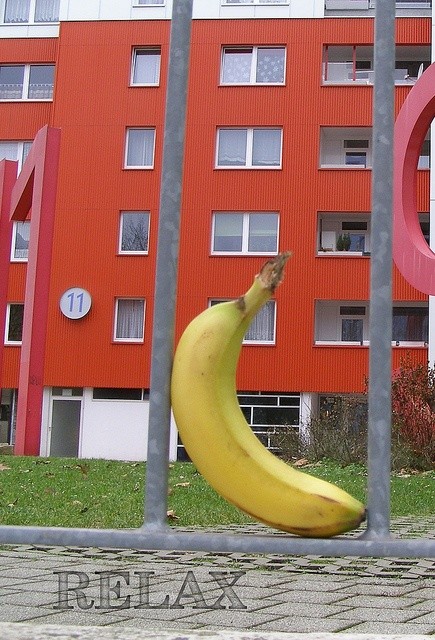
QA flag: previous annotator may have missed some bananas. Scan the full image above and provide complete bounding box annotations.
[170,248,368,540]
